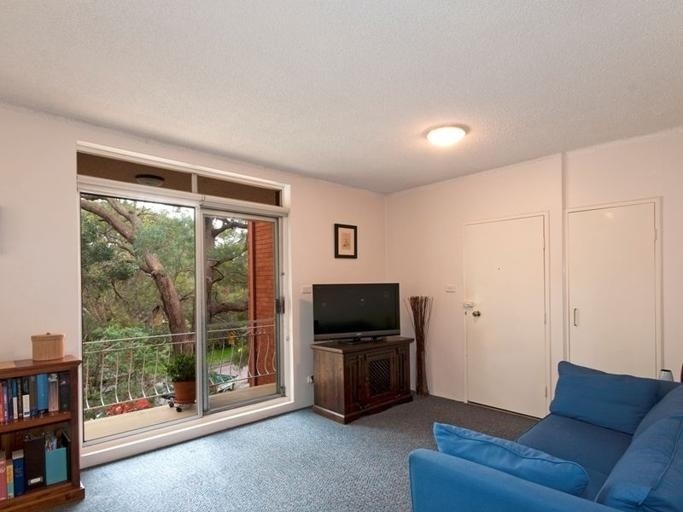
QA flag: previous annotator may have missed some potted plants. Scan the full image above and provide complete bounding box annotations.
[157,349,196,405]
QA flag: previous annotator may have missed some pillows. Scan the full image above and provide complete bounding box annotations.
[550,362,661,435]
[432,421,591,497]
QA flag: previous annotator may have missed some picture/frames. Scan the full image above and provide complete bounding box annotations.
[334,223,358,259]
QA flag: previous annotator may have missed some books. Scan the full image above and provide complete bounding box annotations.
[0,372,72,502]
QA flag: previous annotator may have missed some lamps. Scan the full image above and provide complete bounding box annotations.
[134,174,165,187]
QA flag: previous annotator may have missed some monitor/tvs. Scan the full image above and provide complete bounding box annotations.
[313,283,400,344]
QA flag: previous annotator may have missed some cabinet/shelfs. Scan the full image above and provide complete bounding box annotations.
[310,337,415,424]
[0,355,86,512]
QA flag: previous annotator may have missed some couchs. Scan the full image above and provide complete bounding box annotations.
[407,361,683,512]
